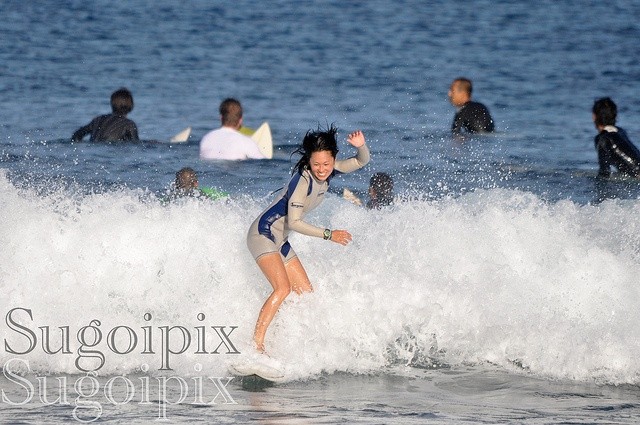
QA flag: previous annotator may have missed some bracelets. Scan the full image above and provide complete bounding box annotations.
[329,230,332,242]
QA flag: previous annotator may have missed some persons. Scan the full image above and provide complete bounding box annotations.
[199,99,266,163]
[246,118,372,356]
[447,76,495,134]
[168,168,202,201]
[365,171,395,209]
[590,97,640,181]
[70,87,139,148]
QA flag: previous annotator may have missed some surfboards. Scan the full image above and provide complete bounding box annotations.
[343,188,363,208]
[252,122,273,159]
[170,128,191,143]
[228,361,286,387]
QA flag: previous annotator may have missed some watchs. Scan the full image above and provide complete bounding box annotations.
[323,228,332,240]
